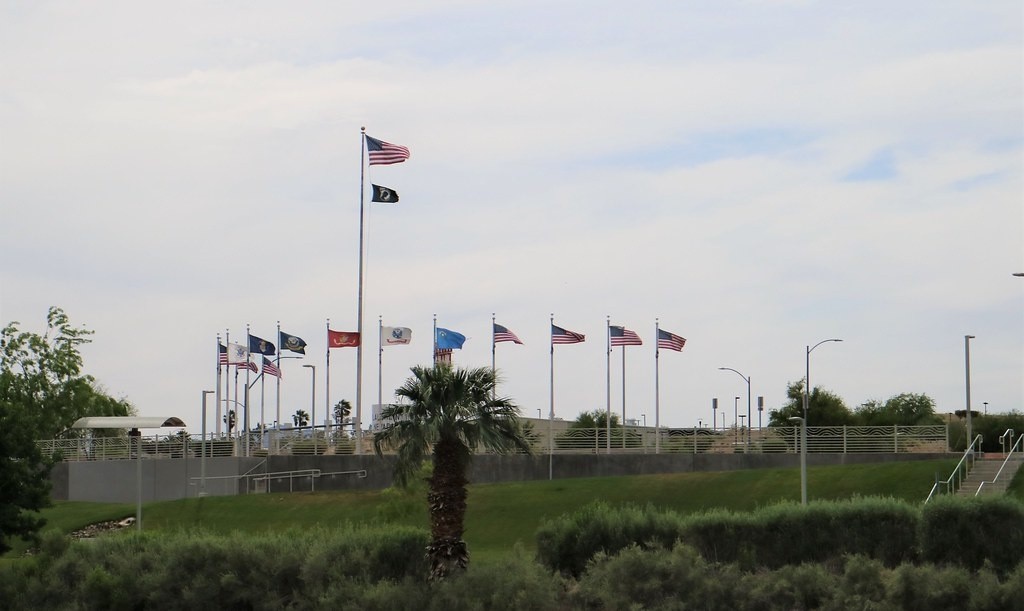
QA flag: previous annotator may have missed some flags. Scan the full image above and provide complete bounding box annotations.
[329,329,360,348]
[610,326,642,346]
[437,328,466,349]
[372,184,399,203]
[280,332,307,355]
[552,324,585,344]
[365,135,410,167]
[220,342,258,374]
[382,326,412,346]
[264,357,281,378]
[658,329,686,352]
[494,324,523,344]
[249,334,276,356]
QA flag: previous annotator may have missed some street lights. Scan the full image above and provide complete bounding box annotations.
[801,339,843,504]
[222,357,303,432]
[719,368,751,445]
